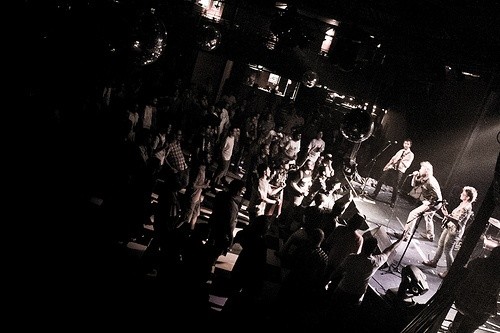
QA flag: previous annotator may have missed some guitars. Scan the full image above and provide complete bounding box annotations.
[383,156,404,170]
[440,198,452,228]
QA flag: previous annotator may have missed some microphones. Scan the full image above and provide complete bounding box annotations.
[388,140,398,144]
[434,199,447,204]
[409,172,419,176]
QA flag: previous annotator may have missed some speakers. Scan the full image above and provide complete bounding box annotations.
[343,197,382,229]
[361,225,397,270]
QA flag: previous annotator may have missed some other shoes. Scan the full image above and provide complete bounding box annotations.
[423,260,437,268]
[394,232,407,242]
[438,272,447,278]
[391,202,395,207]
[423,235,433,242]
[369,194,377,199]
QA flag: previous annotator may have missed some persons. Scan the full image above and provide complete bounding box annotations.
[119,83,410,308]
[367,139,415,208]
[422,186,478,278]
[394,161,442,242]
